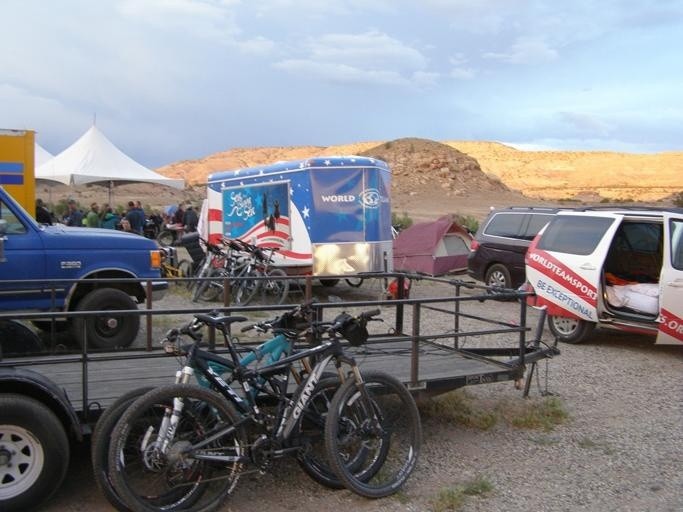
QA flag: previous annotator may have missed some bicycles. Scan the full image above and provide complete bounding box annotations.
[139,211,192,288]
[344,276,363,287]
[185,235,289,305]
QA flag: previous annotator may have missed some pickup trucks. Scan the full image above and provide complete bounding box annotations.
[0,184,169,351]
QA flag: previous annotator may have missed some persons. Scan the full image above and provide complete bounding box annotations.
[34,199,199,236]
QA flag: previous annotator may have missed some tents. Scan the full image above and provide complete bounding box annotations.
[391,216,475,278]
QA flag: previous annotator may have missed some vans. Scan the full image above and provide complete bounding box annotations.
[514,202,682,346]
[197,154,393,291]
[467,204,583,303]
